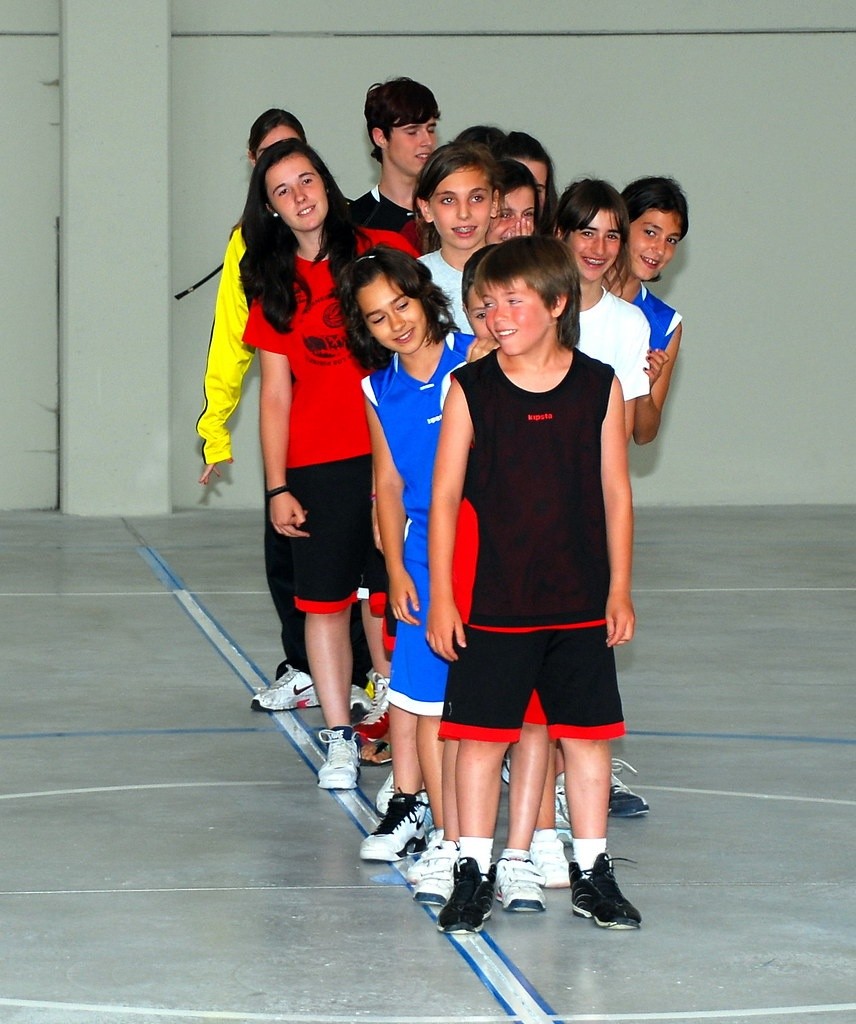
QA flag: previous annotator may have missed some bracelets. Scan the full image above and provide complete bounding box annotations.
[267,486,288,497]
[370,494,377,500]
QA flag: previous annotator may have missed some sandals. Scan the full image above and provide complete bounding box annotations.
[360,726,392,766]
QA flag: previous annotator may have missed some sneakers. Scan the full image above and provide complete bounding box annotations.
[374,769,394,818]
[406,833,442,883]
[349,683,374,726]
[554,785,573,846]
[413,840,462,906]
[435,857,498,935]
[359,787,433,862]
[353,671,392,746]
[533,828,572,888]
[496,859,547,912]
[500,752,510,784]
[251,664,321,712]
[317,726,360,790]
[568,853,643,930]
[607,758,651,817]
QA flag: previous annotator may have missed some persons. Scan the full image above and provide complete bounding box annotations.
[193,74,688,909]
[426,237,644,935]
